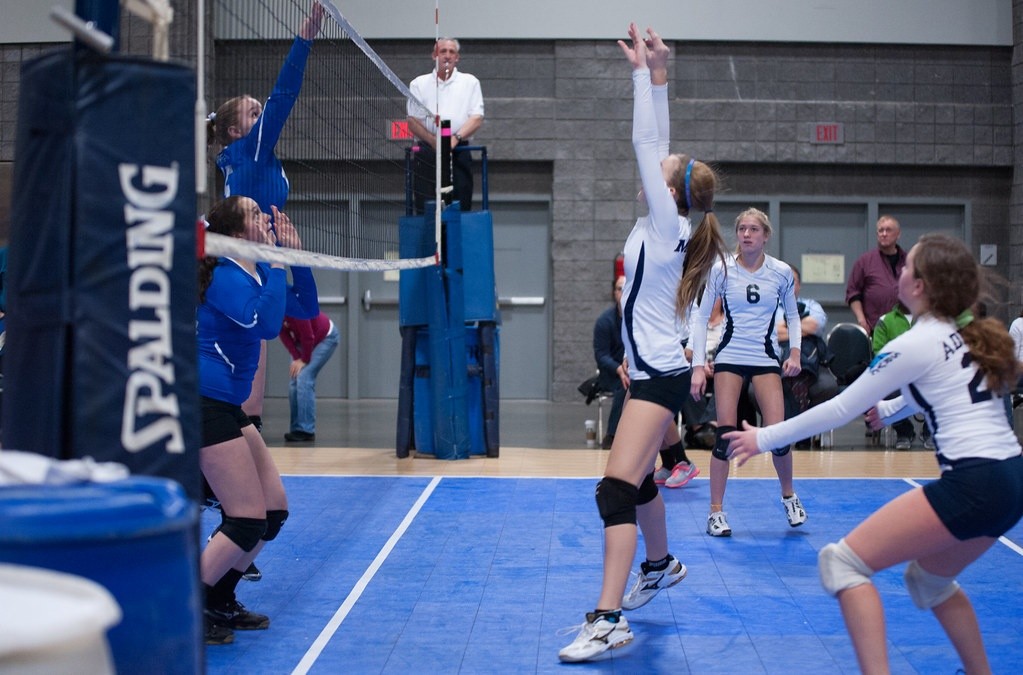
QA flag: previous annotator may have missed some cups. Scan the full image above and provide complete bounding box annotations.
[585,420,597,447]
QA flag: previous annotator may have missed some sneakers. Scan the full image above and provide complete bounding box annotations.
[621,554,687,611]
[555,609,634,662]
[654,466,672,483]
[216,593,270,629]
[202,600,234,646]
[664,461,700,488]
[920,434,937,449]
[895,435,913,449]
[706,512,732,536]
[782,493,806,526]
[207,527,262,582]
[284,430,316,442]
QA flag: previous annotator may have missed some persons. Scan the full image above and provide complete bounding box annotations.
[593,274,631,449]
[654,418,700,488]
[1008,310,1023,360]
[872,299,935,450]
[721,232,1023,675]
[844,215,907,335]
[195,194,319,644]
[678,278,726,448]
[200,0,324,581]
[558,22,728,662]
[279,310,340,442]
[776,264,827,450]
[690,207,807,536]
[406,35,484,214]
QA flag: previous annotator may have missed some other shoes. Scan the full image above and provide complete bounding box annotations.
[601,435,613,448]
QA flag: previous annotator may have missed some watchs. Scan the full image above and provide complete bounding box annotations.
[454,134,461,141]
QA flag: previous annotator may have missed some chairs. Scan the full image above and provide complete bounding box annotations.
[594,320,910,450]
